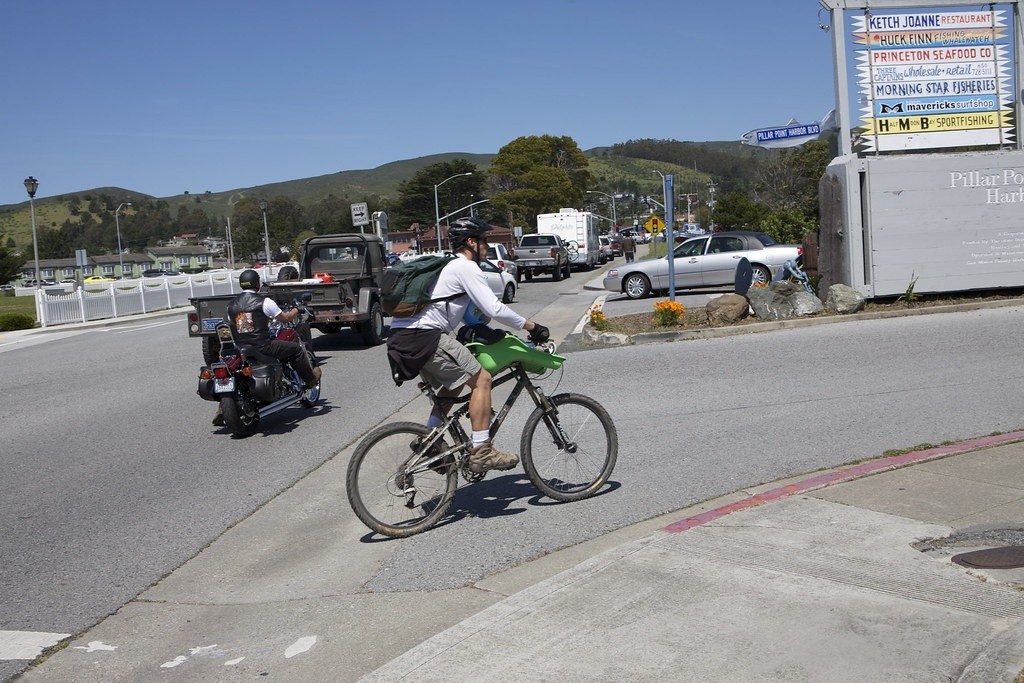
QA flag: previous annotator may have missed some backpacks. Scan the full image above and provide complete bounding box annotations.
[381,252,467,317]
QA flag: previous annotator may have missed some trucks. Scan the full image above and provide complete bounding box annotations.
[537,212,600,272]
[187,232,401,370]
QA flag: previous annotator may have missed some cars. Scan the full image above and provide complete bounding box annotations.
[477,243,520,304]
[0,277,117,292]
[144,269,179,278]
[602,230,804,299]
[595,229,665,264]
[252,260,298,269]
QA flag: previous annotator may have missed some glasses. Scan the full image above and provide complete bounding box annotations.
[479,237,488,243]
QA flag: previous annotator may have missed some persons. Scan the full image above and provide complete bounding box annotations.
[621,230,636,263]
[641,232,645,244]
[386,217,550,473]
[212,270,322,426]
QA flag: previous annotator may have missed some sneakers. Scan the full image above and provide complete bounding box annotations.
[410,435,444,472]
[306,367,321,389]
[469,437,520,472]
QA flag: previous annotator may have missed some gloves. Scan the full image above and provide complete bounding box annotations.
[527,322,550,346]
[295,306,305,314]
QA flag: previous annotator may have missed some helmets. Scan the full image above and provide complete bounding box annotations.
[447,217,494,245]
[239,270,260,290]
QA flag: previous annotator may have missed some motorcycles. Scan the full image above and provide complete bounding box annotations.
[195,292,322,438]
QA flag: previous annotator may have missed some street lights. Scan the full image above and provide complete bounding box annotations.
[260,201,273,276]
[22,175,41,291]
[651,170,667,220]
[115,203,132,265]
[435,172,472,253]
[586,190,617,225]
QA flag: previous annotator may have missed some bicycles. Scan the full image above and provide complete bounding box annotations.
[344,330,619,539]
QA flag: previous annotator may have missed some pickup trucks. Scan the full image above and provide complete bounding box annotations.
[513,233,571,281]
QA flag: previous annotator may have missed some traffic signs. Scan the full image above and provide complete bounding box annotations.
[351,202,369,226]
